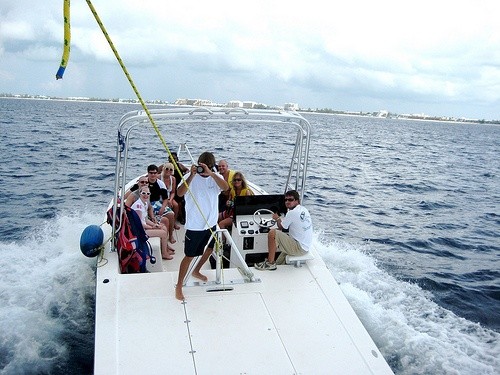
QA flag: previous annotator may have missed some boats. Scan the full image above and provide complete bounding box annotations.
[80,105,395,374]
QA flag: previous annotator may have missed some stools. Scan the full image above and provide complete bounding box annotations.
[285,255,312,268]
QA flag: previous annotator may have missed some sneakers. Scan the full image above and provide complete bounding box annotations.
[254,258,277,270]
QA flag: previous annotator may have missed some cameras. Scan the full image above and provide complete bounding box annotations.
[195,166,204,173]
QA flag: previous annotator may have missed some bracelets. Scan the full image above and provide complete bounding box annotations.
[275,217,280,221]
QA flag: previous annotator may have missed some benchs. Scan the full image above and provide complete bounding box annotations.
[131,236,164,273]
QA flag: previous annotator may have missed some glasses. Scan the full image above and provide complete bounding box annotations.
[166,168,172,171]
[169,157,177,160]
[139,181,149,184]
[148,171,158,174]
[285,198,295,201]
[141,191,151,196]
[233,179,241,181]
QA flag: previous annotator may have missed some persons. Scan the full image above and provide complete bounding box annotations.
[175,152,230,300]
[124,165,176,244]
[254,190,313,271]
[156,163,180,230]
[218,172,250,229]
[130,186,175,260]
[218,160,236,213]
[168,152,190,225]
[125,176,175,252]
